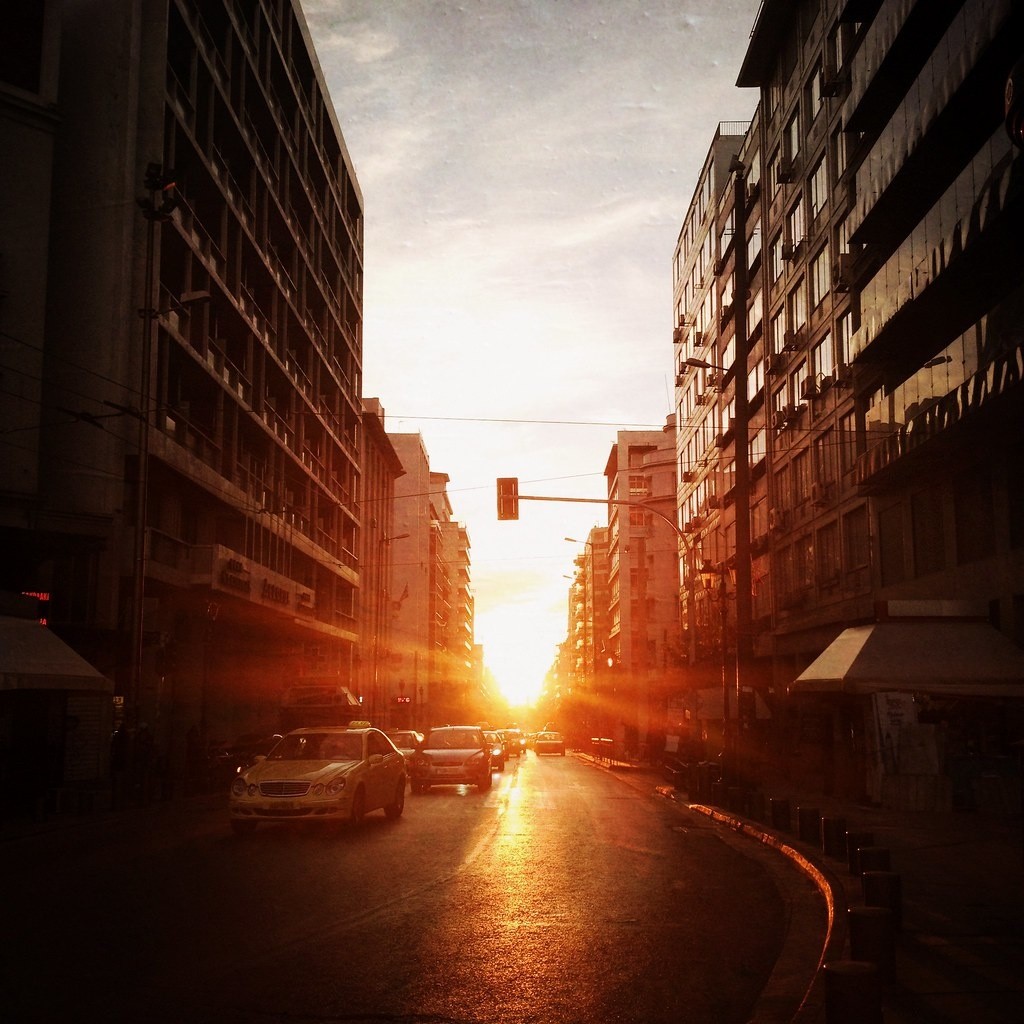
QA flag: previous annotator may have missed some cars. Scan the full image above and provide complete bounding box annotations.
[382,730,421,774]
[529,732,566,757]
[483,730,527,770]
[231,720,405,831]
[408,727,493,789]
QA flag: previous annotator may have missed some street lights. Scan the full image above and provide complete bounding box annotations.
[566,536,602,761]
[563,574,588,755]
[372,534,410,729]
